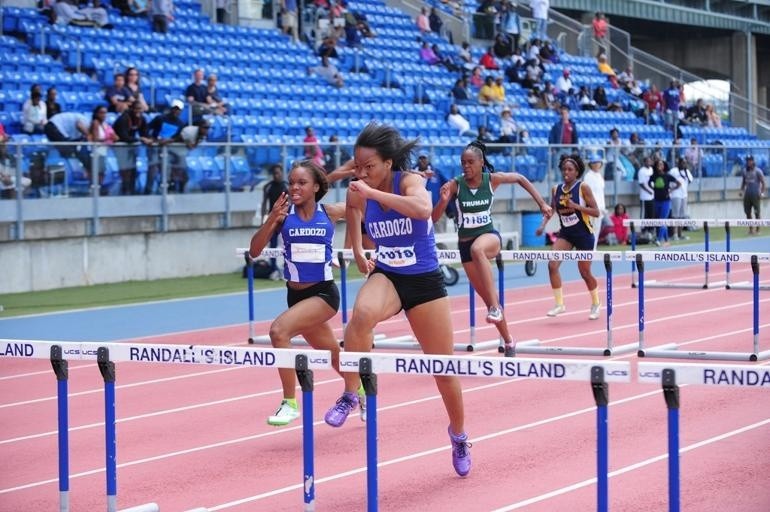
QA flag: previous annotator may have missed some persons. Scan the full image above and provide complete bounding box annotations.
[429,141,555,359]
[33,0,178,36]
[322,122,473,479]
[215,0,228,24]
[259,125,378,283]
[534,154,603,322]
[413,1,767,252]
[262,0,375,89]
[0,67,231,203]
[249,157,369,425]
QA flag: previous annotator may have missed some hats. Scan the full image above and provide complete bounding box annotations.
[502,106,511,112]
[745,154,752,159]
[171,99,183,111]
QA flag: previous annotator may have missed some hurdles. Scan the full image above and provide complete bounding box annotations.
[346,248,502,352]
[338,350,630,511]
[622,218,716,289]
[493,249,640,358]
[638,360,770,512]
[716,218,770,290]
[236,246,388,348]
[624,250,770,362]
[0,337,333,512]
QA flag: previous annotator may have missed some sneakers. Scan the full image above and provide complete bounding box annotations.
[486,305,504,322]
[324,392,359,426]
[589,303,600,319]
[358,392,366,421]
[267,400,300,425]
[448,424,471,475]
[547,304,565,315]
[504,335,518,357]
